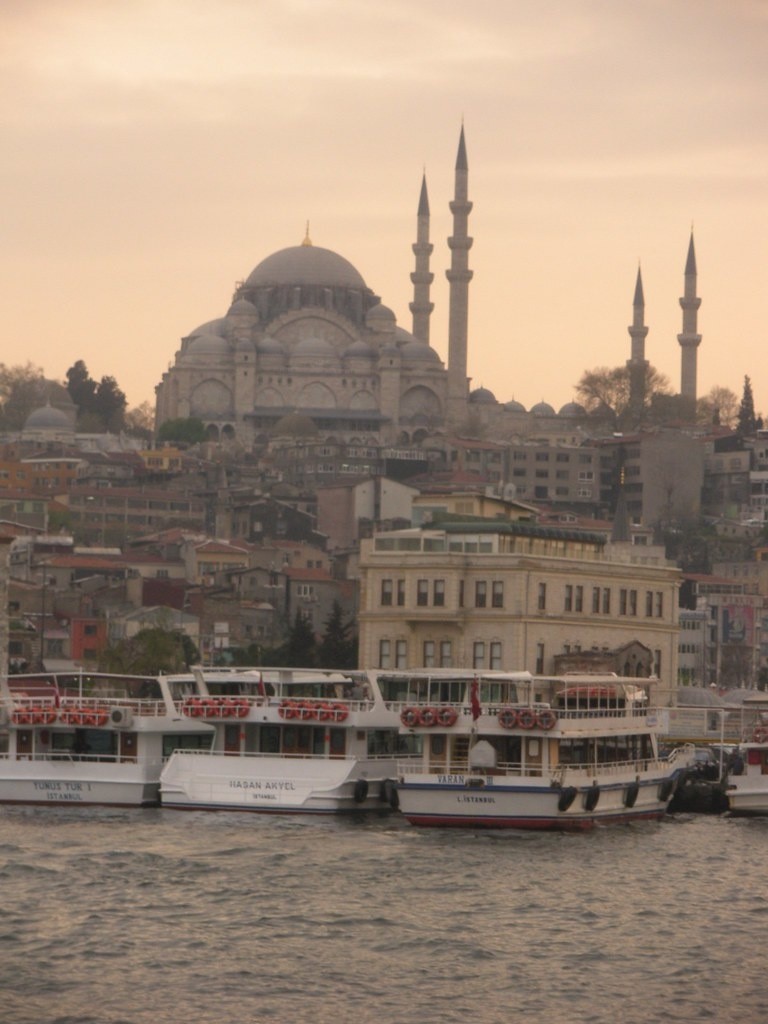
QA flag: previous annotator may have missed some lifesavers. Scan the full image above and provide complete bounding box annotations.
[556,776,675,813]
[182,698,250,719]
[10,705,109,726]
[353,777,401,812]
[278,700,351,723]
[497,707,558,732]
[399,705,458,728]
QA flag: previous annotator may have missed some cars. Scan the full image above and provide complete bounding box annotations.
[658,742,745,781]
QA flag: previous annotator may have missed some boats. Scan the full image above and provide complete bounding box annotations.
[726,712,768,823]
[0,666,696,830]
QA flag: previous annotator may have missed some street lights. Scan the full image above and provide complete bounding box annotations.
[718,709,730,785]
